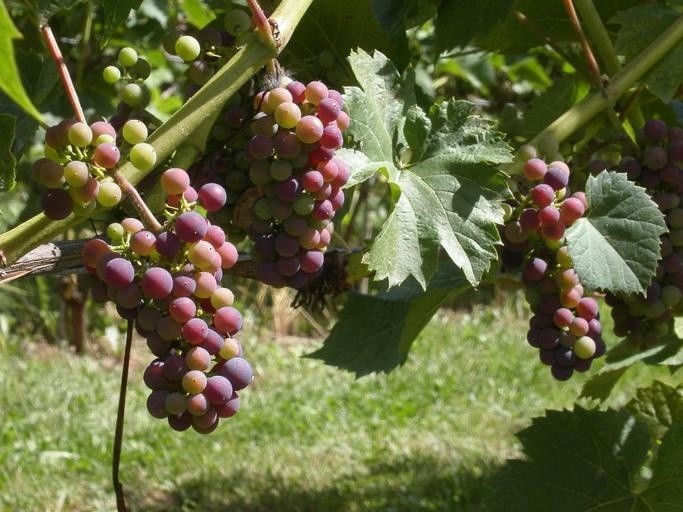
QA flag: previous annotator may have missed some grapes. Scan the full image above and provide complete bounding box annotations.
[30,11,683,436]
